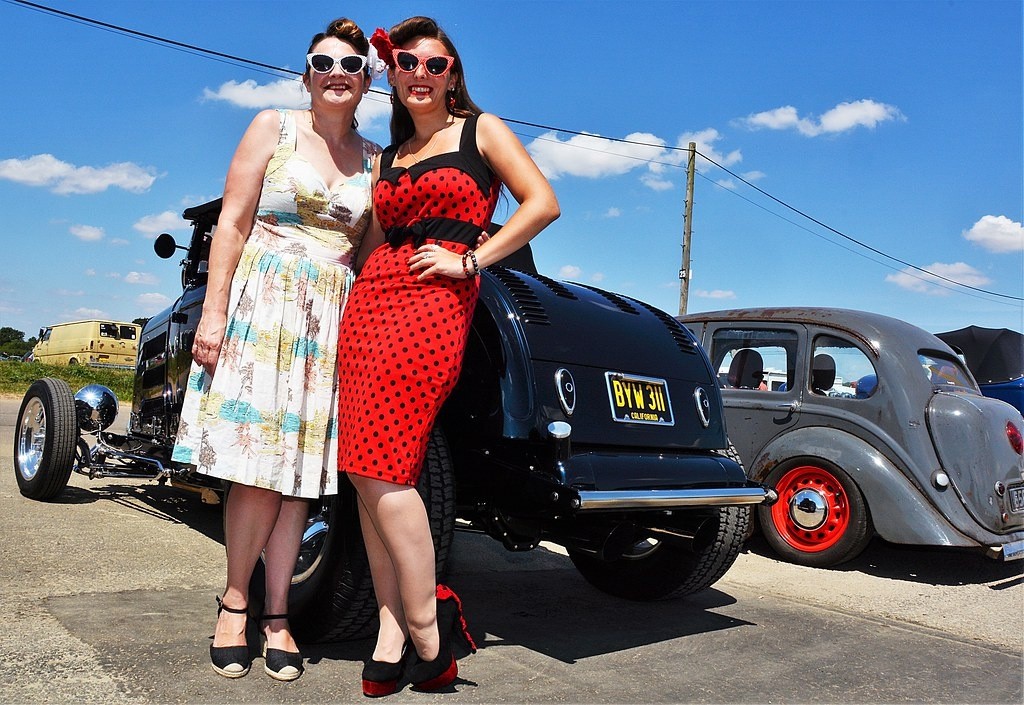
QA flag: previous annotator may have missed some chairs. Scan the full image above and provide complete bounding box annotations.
[812,354,836,397]
[727,349,764,390]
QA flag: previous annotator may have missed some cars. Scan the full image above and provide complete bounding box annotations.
[672,306,1024,568]
[854,325,1024,417]
[0,349,34,363]
[12,196,779,653]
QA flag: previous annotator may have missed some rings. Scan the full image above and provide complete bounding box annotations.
[192,343,198,348]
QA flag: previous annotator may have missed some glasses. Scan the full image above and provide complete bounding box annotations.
[306,53,367,74]
[392,49,455,77]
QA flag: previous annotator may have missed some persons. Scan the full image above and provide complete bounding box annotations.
[336,17,561,697]
[758,379,768,391]
[191,17,491,683]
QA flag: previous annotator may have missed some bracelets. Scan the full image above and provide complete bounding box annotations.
[462,249,482,279]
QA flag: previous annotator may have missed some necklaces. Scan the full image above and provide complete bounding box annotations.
[408,128,442,163]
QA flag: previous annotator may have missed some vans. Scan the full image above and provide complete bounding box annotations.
[31,320,141,373]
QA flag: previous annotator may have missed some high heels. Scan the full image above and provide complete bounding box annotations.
[408,583,475,689]
[257,606,303,680]
[362,633,411,696]
[209,595,251,677]
